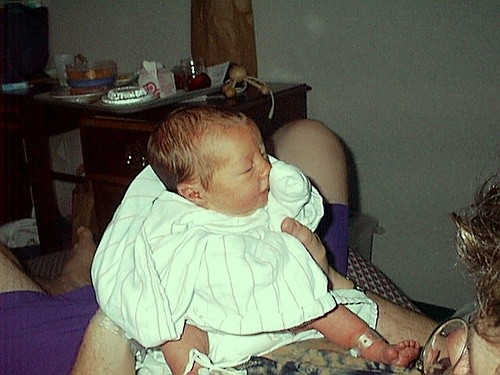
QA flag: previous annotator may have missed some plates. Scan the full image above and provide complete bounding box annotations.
[49,91,103,103]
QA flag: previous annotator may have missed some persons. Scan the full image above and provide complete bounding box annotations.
[0,120,500,375]
[91,103,421,375]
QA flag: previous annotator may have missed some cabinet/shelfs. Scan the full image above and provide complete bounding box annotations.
[17,78,311,256]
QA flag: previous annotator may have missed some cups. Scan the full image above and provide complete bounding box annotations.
[181,57,205,91]
[54,54,75,88]
